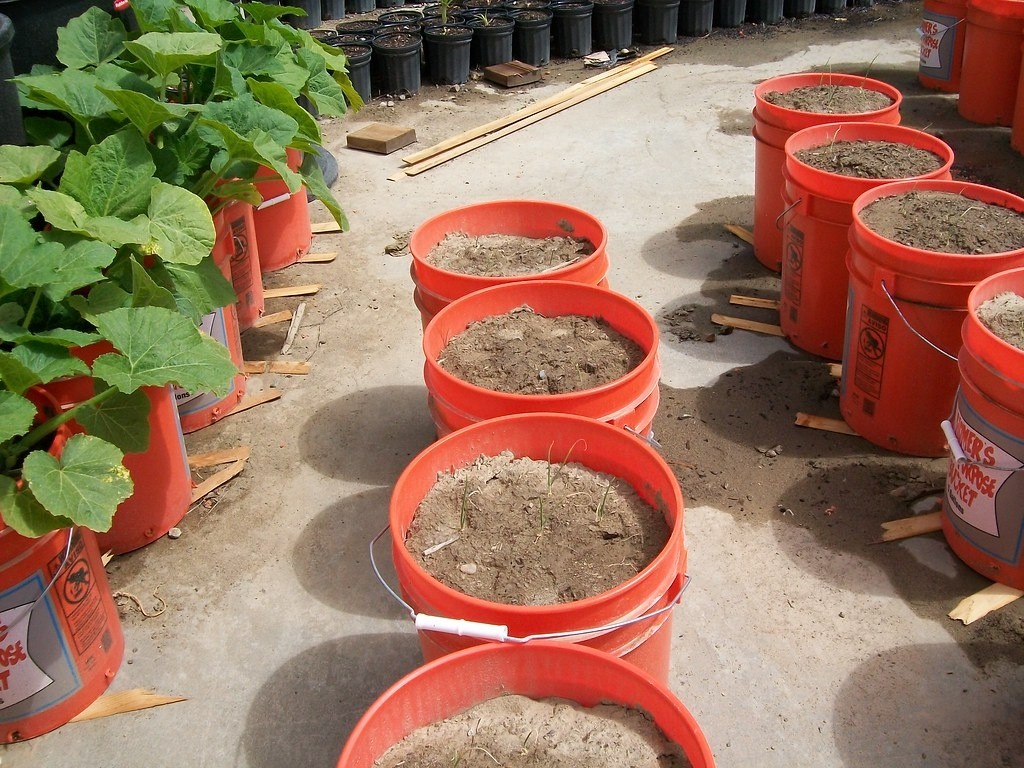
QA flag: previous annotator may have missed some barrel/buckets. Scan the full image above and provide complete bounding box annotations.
[325,198,714,764]
[748,72,1024,588]
[918,3,1024,149]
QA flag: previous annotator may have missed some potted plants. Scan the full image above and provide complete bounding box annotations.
[0,2,886,753]
